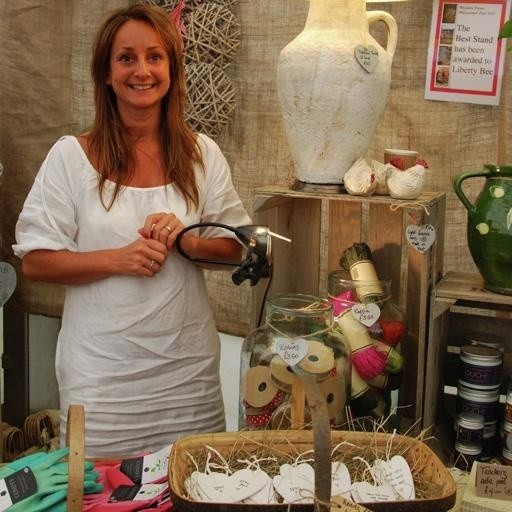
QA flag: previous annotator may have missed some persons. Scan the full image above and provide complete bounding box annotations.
[11,3,253,458]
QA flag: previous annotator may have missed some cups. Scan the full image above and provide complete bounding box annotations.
[384,149,418,172]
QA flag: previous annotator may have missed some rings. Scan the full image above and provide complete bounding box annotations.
[149,260,155,269]
[165,225,172,233]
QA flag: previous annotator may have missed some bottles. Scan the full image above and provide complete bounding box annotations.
[454,412,482,470]
[238,293,352,433]
[317,270,407,433]
[454,164,510,295]
